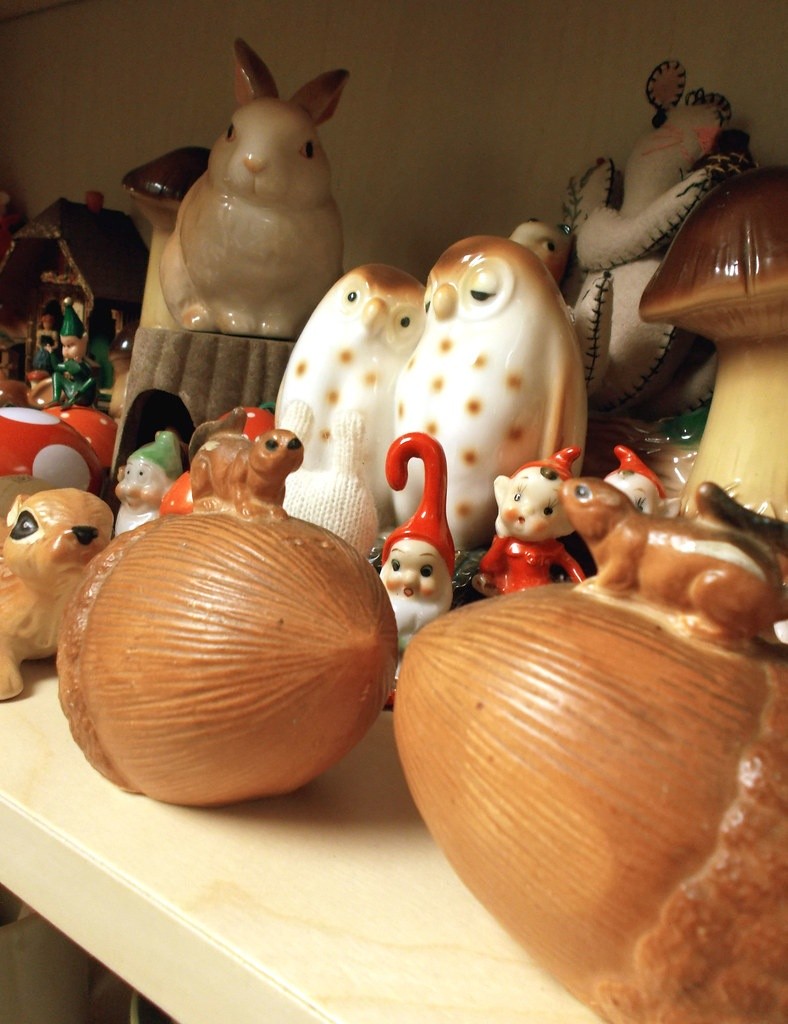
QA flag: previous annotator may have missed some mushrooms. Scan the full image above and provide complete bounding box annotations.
[637,162,788,525]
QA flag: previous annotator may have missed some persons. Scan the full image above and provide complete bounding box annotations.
[45,297,96,409]
[480,446,585,597]
[379,433,456,708]
[36,314,58,353]
[114,431,182,535]
[603,447,681,520]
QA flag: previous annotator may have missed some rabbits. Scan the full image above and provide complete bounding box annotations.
[158,36,352,341]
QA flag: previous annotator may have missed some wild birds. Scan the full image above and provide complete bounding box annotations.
[272,236,587,563]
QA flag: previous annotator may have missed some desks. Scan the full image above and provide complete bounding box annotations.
[0,668,610,1024]
